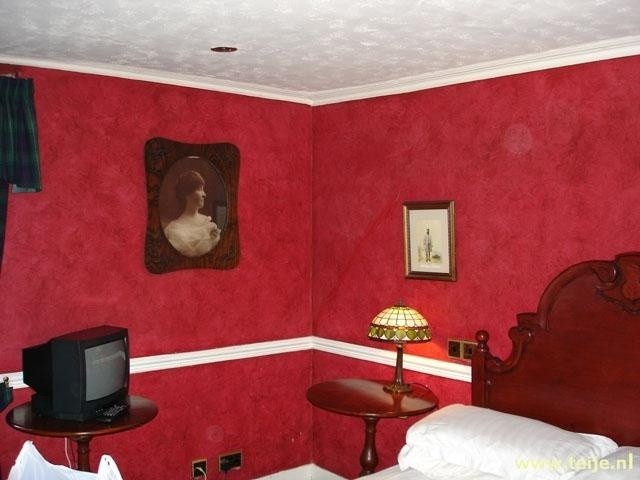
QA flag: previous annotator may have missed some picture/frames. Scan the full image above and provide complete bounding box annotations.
[144,137,240,274]
[402,199,457,282]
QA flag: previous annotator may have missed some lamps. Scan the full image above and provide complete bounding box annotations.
[367,305,432,393]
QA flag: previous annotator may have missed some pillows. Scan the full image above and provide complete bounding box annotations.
[397,403,640,480]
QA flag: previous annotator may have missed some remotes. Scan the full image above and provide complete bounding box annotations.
[100,403,131,423]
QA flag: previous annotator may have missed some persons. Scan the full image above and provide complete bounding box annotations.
[165,170,221,256]
[423,229,432,265]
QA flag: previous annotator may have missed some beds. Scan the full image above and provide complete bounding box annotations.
[352,251,640,480]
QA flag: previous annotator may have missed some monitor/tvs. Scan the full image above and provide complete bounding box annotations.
[21,325,130,422]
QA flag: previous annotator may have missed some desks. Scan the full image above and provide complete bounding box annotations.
[306,377,438,478]
[5,394,158,472]
